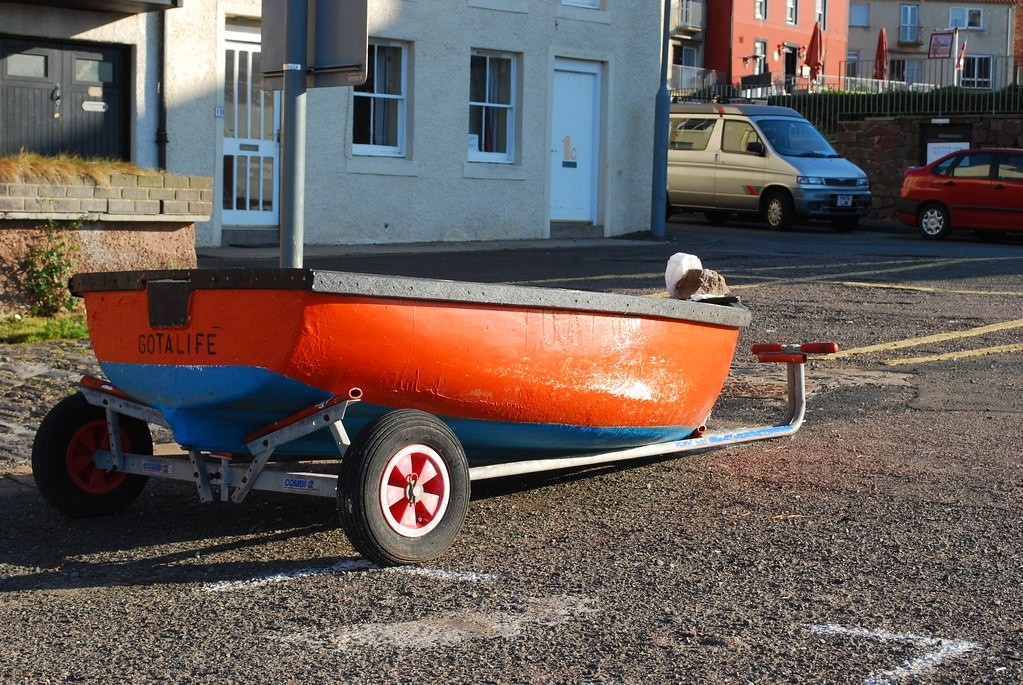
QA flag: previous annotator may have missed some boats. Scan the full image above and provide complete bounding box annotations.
[70,267,752,459]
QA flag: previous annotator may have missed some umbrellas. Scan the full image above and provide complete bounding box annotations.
[873,28,888,80]
[803,21,825,93]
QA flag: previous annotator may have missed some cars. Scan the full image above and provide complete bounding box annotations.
[896,147,1023,242]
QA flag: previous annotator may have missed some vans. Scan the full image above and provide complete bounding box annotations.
[668,102,872,233]
